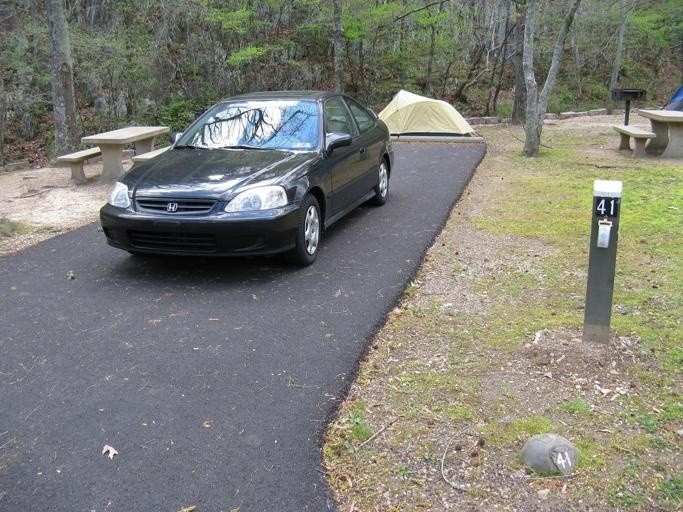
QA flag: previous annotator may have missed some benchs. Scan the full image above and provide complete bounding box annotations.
[132,147,170,165]
[611,121,655,155]
[56,146,98,182]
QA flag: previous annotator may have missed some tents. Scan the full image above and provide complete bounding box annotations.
[377,89,474,135]
[661,85,683,111]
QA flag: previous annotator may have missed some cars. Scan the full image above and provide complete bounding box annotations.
[100,91,394,267]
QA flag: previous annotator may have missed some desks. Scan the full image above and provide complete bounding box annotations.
[638,108,682,156]
[80,127,170,177]
